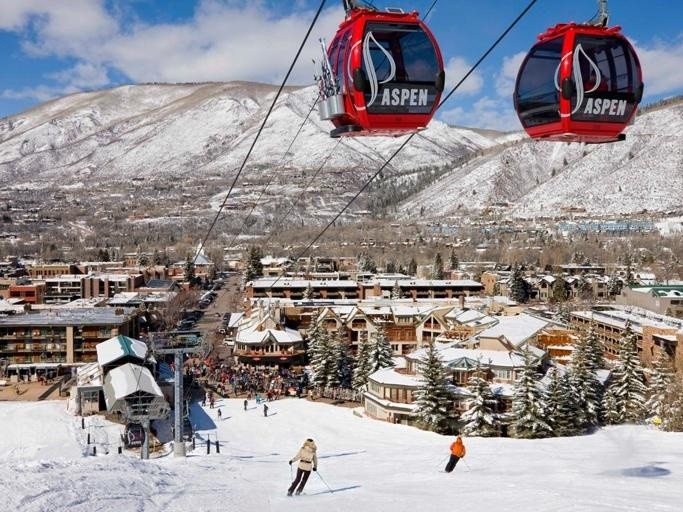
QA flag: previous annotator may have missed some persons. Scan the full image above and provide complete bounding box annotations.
[169,350,286,421]
[288,439,318,496]
[584,67,609,91]
[445,437,465,471]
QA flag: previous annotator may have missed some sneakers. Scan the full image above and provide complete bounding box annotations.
[288,490,292,496]
[296,490,299,495]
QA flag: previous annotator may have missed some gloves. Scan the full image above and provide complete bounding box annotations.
[289,461,292,464]
[313,468,317,471]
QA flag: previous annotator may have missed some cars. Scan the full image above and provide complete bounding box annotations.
[213,312,235,346]
[176,290,217,331]
